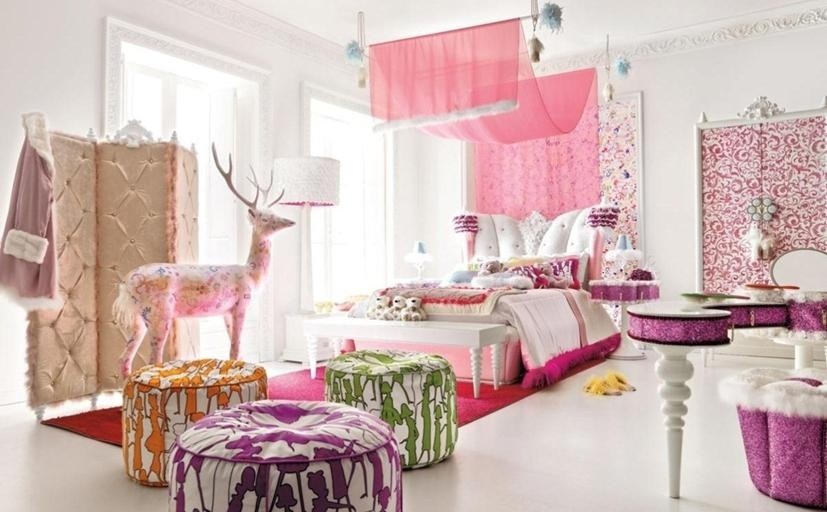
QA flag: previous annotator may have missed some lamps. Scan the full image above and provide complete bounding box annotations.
[607,234,642,280]
[405,240,429,282]
[274,152,340,365]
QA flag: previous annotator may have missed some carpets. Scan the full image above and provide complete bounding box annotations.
[37,361,540,449]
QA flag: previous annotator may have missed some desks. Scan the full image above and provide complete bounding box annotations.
[623,292,797,501]
[759,292,827,379]
[588,277,660,362]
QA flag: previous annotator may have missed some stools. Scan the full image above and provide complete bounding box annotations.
[730,369,826,512]
[168,396,405,511]
[115,358,269,490]
[324,348,460,471]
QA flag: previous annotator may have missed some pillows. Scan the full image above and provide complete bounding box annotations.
[447,250,589,291]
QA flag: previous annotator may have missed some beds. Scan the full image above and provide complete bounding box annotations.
[338,195,621,392]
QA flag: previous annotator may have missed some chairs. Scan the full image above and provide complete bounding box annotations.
[770,245,827,289]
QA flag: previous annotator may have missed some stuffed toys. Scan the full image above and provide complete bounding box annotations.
[478,260,508,276]
[534,262,572,289]
[381,296,407,319]
[401,297,427,322]
[366,296,390,317]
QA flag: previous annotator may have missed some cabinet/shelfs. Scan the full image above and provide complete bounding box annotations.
[692,95,827,368]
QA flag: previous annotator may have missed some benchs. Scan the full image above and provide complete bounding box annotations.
[304,318,510,400]
